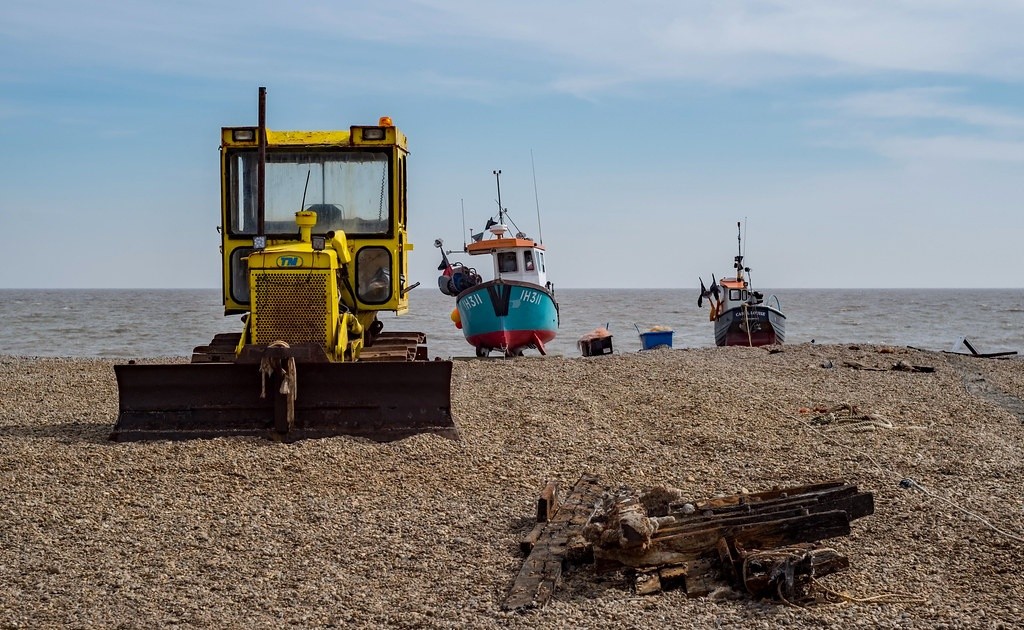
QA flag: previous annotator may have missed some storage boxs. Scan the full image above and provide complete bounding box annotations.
[580,335,613,358]
[639,331,673,349]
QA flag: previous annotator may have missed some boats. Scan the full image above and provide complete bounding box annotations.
[433,169,561,357]
[697,215,787,348]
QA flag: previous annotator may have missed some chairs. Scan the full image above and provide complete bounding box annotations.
[299,203,346,233]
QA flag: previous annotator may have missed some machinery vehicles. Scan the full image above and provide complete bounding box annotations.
[108,86,456,435]
